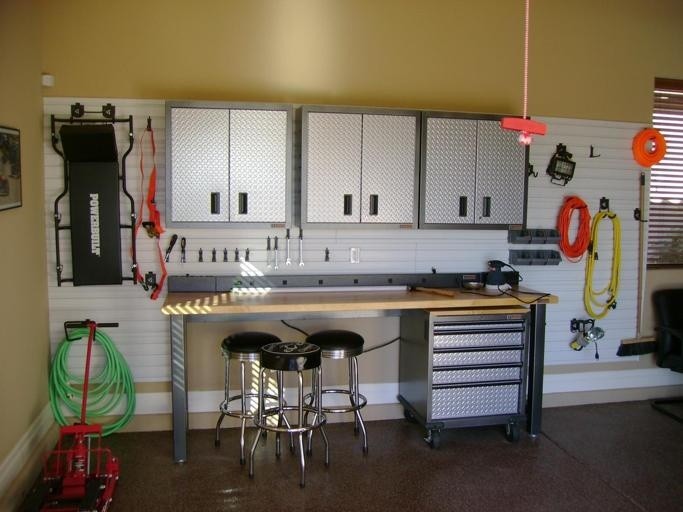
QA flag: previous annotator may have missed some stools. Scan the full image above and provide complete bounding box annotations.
[215,330,372,489]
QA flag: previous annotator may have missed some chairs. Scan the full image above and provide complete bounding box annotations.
[648,289,683,426]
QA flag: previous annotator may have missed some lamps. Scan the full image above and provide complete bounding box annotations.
[500,0,576,187]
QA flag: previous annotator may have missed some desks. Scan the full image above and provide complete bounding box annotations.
[159,291,558,464]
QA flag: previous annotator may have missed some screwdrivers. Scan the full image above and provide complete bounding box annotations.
[163,235,177,264]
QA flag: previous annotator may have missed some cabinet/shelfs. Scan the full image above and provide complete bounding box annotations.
[166,100,292,230]
[418,109,530,232]
[294,104,422,230]
[400,311,529,450]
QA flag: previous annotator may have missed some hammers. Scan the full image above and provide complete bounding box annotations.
[408,285,456,296]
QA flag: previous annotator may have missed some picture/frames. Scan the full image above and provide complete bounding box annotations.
[0,126,24,212]
